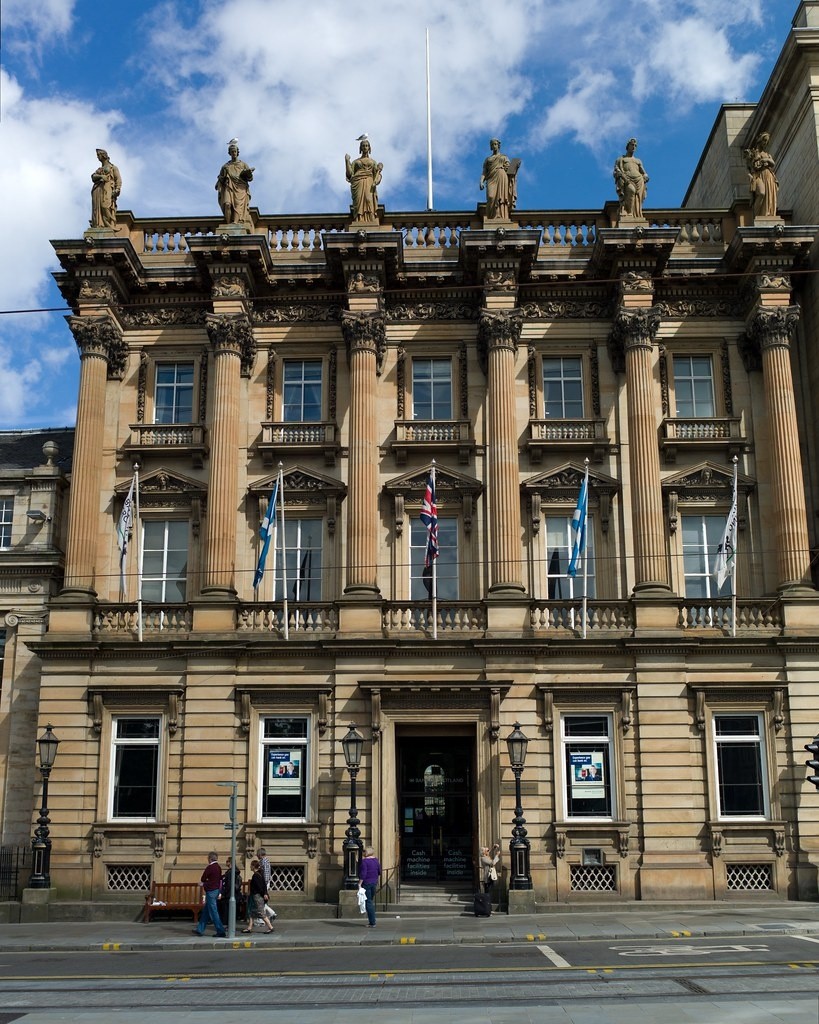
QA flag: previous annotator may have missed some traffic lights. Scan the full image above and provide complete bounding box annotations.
[804,738,819,789]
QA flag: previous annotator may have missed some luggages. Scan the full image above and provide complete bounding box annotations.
[474,881,492,917]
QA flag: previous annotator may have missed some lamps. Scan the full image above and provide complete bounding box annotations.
[29,510,46,522]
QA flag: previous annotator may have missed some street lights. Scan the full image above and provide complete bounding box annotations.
[215,784,239,938]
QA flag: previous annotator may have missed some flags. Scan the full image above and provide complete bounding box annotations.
[567,472,588,578]
[712,473,737,589]
[116,477,133,598]
[253,479,277,588]
[420,472,440,567]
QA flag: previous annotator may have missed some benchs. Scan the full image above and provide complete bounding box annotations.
[144,881,203,924]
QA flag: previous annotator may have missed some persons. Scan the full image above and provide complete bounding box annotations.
[585,765,602,781]
[219,857,244,930]
[345,140,382,221]
[358,846,380,928]
[256,848,276,926]
[282,762,298,778]
[90,149,122,232]
[480,844,500,913]
[242,860,274,933]
[614,137,647,216]
[480,138,521,219]
[192,852,226,937]
[747,133,779,216]
[215,145,254,225]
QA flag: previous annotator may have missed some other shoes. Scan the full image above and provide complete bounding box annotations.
[263,928,275,934]
[212,933,226,937]
[270,914,278,922]
[241,928,252,933]
[191,929,203,936]
[366,921,376,927]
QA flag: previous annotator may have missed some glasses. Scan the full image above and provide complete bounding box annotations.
[487,850,489,851]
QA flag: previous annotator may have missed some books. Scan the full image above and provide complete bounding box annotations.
[506,157,520,174]
